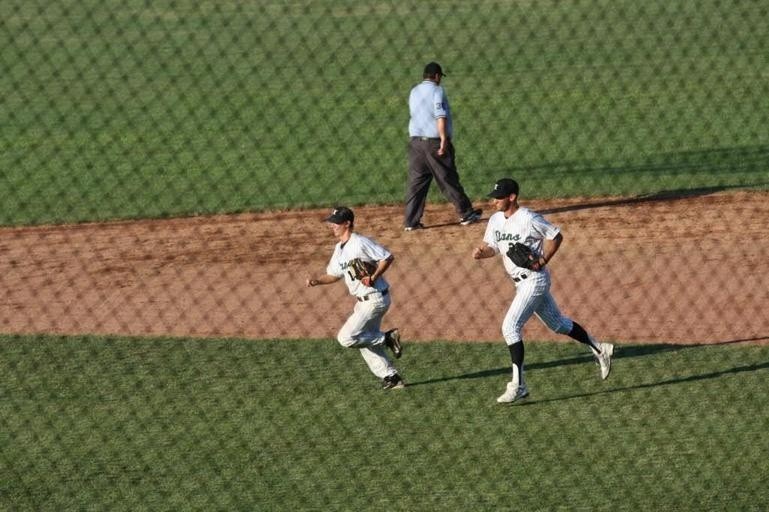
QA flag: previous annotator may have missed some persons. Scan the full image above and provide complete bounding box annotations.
[472,178,614,405]
[402,61,483,232]
[305,206,406,392]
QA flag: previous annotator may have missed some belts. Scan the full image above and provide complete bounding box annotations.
[412,136,440,141]
[512,273,528,282]
[356,287,389,302]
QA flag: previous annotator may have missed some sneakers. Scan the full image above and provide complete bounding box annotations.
[496,382,529,403]
[382,375,405,390]
[404,223,424,231]
[595,342,615,380]
[459,208,483,226]
[384,327,403,359]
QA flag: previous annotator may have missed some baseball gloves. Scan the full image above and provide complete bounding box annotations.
[348,258,375,284]
[504,241,541,270]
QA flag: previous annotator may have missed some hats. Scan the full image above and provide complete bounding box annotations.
[486,177,520,199]
[423,61,447,77]
[320,206,354,225]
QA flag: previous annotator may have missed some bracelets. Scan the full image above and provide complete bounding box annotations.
[540,256,547,262]
